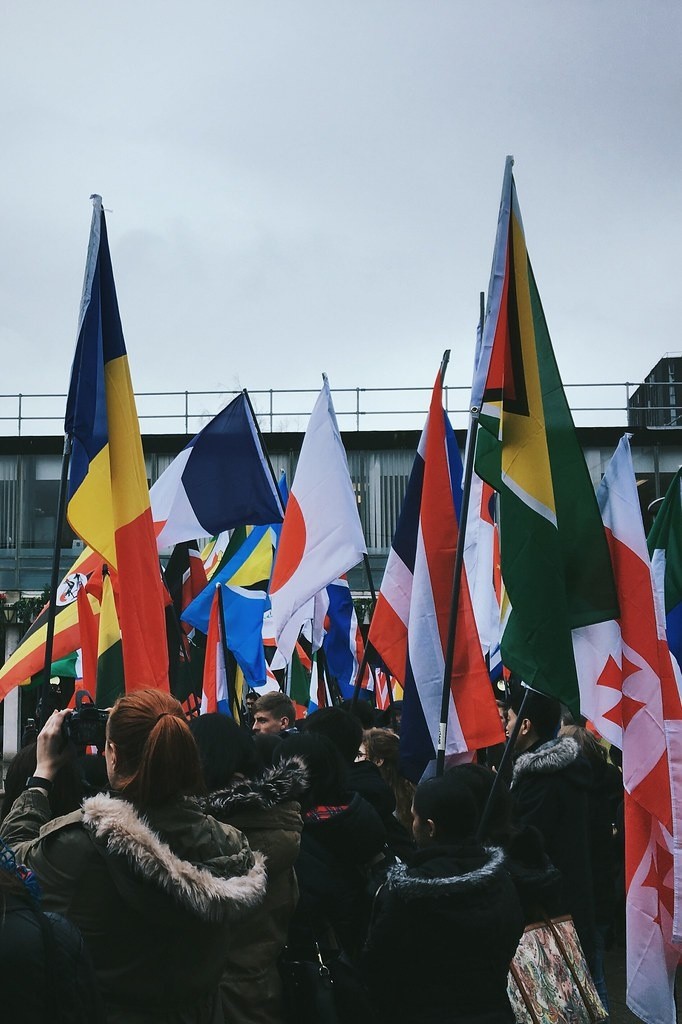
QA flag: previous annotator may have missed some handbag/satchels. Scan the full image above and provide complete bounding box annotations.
[505,908,609,1024]
[308,949,376,1024]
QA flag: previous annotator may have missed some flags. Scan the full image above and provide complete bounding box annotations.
[36,193,170,734]
[577,431,682,1024]
[0,367,681,720]
[436,155,623,777]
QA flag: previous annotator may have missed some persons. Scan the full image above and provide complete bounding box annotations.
[0,864,59,1024]
[2,695,623,850]
[353,777,525,1024]
[353,727,418,849]
[0,688,267,1023]
[274,731,389,967]
[489,692,609,1024]
[180,712,305,1024]
[445,765,562,927]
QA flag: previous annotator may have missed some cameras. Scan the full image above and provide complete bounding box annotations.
[63,714,110,752]
